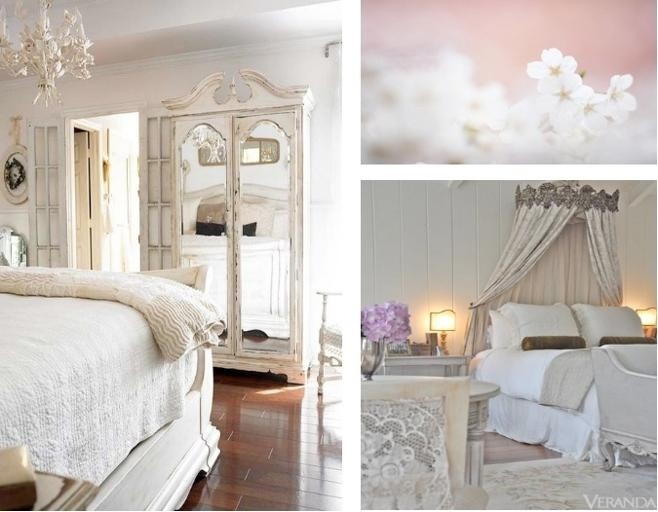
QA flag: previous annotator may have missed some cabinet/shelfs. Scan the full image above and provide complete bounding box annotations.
[166,72,315,386]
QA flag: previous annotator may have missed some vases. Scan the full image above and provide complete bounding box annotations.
[360,336,384,381]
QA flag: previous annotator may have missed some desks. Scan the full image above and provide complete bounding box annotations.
[362,375,501,485]
[383,352,470,376]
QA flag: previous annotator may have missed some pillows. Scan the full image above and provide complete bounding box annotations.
[195,204,225,223]
[193,222,224,235]
[487,310,510,348]
[572,302,644,345]
[243,223,258,237]
[597,335,656,344]
[501,302,580,345]
[522,335,585,349]
[240,200,276,238]
[357,396,453,511]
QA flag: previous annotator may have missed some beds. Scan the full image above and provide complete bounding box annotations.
[1,263,221,512]
[471,303,656,455]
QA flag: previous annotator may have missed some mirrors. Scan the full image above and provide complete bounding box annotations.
[173,123,228,345]
[237,120,292,346]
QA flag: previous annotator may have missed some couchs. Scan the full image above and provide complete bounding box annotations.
[591,344,655,474]
[360,379,487,509]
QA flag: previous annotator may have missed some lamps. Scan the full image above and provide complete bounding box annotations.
[1,0,98,113]
[429,310,457,356]
[639,307,657,338]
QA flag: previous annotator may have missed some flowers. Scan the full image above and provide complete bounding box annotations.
[362,300,412,364]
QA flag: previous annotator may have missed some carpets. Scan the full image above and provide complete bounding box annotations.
[481,457,657,510]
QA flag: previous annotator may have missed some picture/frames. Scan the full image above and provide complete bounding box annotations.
[384,338,411,356]
